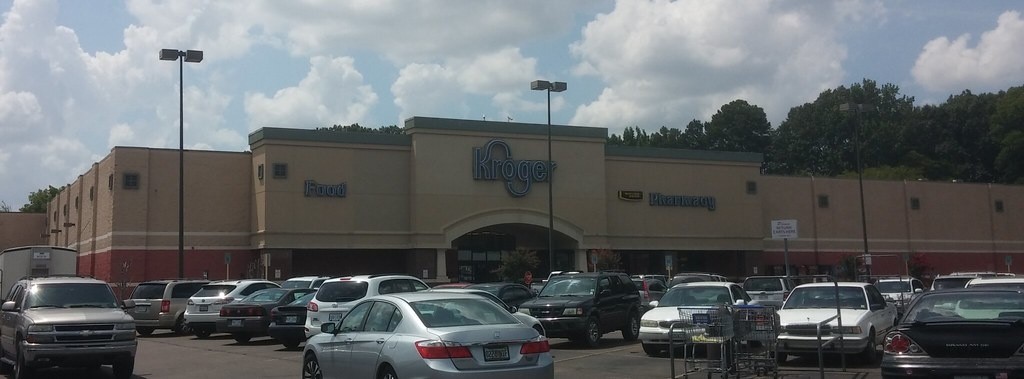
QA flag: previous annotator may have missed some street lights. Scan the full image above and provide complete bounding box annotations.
[157,48,202,280]
[838,102,876,284]
[530,80,569,272]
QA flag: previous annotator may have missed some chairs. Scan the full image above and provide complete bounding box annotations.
[716,294,730,304]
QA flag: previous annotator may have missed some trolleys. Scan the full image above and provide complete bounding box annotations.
[674,305,780,379]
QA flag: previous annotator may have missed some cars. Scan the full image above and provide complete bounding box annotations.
[777,282,898,366]
[266,291,317,350]
[875,289,1024,379]
[301,290,554,379]
[635,280,753,357]
[283,271,1024,323]
[220,287,313,341]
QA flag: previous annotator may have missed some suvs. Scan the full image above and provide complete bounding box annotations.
[125,281,215,337]
[519,271,642,349]
[305,273,431,339]
[0,274,138,379]
[181,279,283,337]
